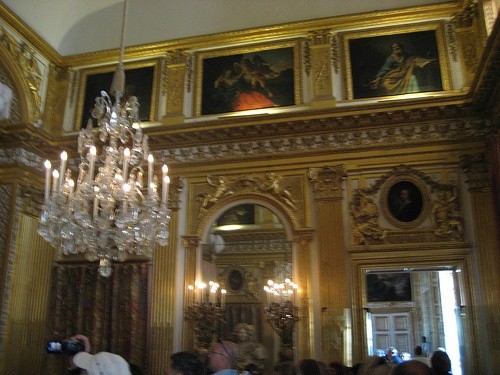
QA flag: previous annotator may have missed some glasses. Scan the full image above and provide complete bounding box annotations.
[216,338,231,358]
[209,350,226,358]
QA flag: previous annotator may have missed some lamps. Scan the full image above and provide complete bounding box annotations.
[264,278,299,344]
[184,281,226,346]
[37,0,171,278]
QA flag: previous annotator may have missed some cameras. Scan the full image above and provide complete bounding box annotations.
[46,340,85,355]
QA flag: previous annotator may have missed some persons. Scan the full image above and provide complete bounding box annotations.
[63,334,131,375]
[131,336,452,375]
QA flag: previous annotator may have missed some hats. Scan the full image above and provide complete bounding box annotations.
[73,350,131,375]
